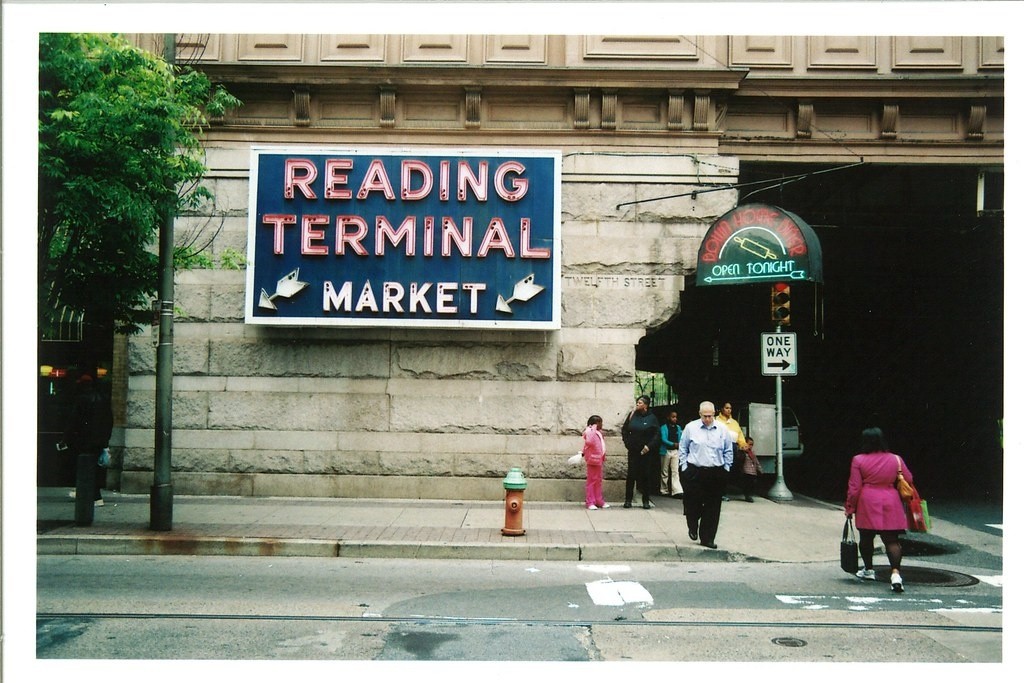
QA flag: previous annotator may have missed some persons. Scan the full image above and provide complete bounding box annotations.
[678,401,733,549]
[716,401,749,501]
[58,360,114,507]
[845,427,913,593]
[621,395,662,509]
[743,436,763,502]
[660,409,684,499]
[581,415,610,510]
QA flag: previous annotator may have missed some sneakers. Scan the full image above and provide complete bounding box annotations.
[890,573,905,592]
[855,568,875,580]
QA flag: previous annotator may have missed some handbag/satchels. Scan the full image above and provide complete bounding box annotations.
[840,517,860,574]
[894,454,914,502]
[906,499,931,533]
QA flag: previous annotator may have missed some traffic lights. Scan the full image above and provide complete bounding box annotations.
[771,282,791,327]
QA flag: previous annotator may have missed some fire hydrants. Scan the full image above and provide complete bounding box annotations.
[501,468,528,537]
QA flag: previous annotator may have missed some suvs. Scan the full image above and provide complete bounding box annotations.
[734,404,804,458]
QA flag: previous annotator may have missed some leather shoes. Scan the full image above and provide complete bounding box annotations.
[689,530,697,540]
[700,540,717,549]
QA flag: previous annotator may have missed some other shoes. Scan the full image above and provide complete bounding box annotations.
[673,494,683,499]
[643,500,650,509]
[623,501,631,508]
[586,505,598,510]
[722,496,730,501]
[596,503,610,508]
[745,497,754,502]
[662,494,672,497]
